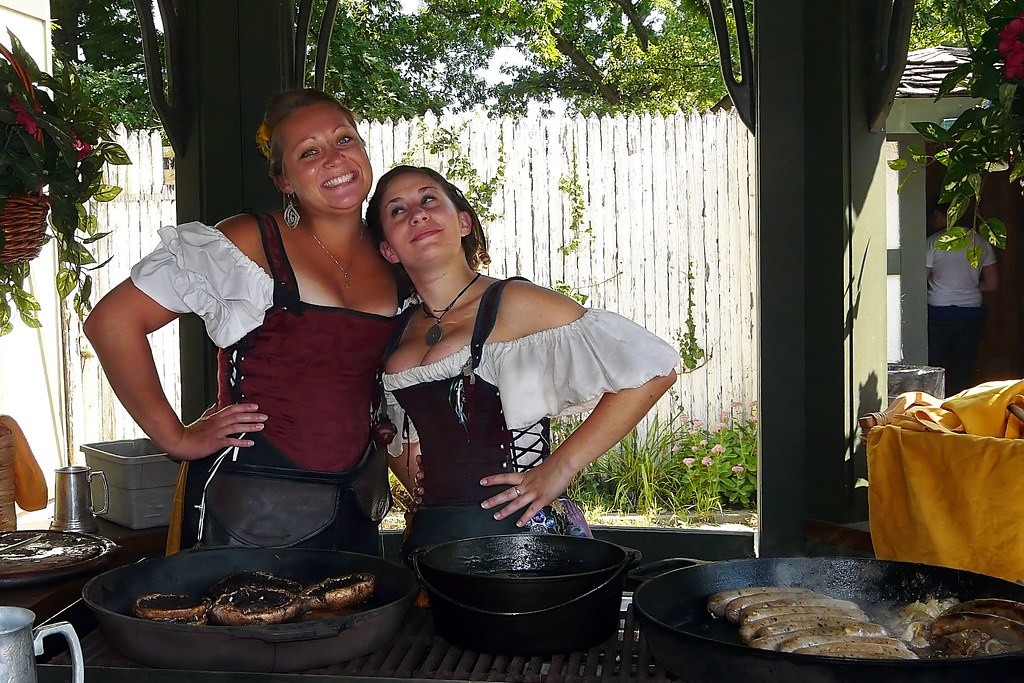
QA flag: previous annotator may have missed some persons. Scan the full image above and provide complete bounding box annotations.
[82,89,411,559]
[367,164,679,562]
[926,197,999,399]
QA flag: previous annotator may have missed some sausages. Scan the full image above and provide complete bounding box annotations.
[706,587,920,659]
[928,598,1024,650]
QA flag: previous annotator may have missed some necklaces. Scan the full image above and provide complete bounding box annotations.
[412,272,480,346]
[299,219,364,290]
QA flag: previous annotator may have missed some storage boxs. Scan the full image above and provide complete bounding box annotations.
[80,437,183,529]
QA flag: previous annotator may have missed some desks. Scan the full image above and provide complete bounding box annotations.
[94,508,203,567]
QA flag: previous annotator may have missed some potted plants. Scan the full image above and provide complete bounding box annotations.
[0,25,132,340]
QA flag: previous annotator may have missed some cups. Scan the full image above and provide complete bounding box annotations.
[50,466,109,533]
[0,606,85,683]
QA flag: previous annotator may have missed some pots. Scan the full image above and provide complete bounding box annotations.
[82,545,421,674]
[632,556,1024,683]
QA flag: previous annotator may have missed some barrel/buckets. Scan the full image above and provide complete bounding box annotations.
[409,532,641,650]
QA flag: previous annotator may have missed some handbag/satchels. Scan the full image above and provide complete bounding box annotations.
[199,442,392,555]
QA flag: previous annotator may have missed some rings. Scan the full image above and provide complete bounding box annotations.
[514,485,522,497]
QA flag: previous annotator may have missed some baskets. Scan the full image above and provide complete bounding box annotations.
[0,42,51,263]
